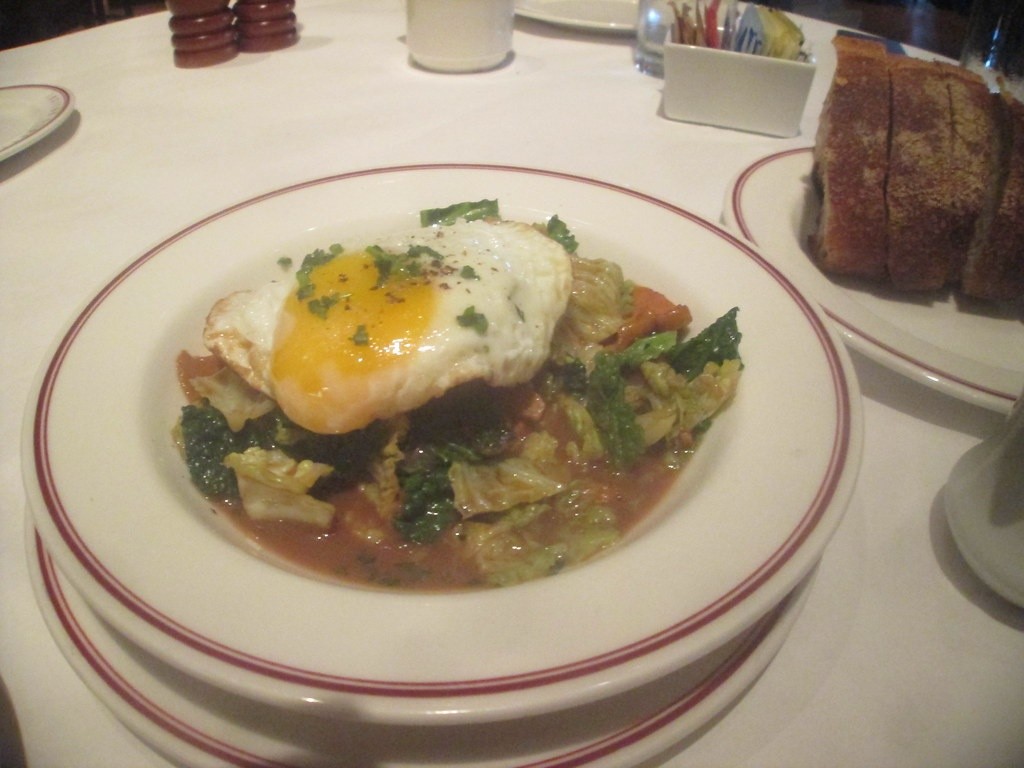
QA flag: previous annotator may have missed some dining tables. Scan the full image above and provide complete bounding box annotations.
[0,0,1024,768]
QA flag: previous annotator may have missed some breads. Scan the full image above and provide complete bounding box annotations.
[805,31,1024,303]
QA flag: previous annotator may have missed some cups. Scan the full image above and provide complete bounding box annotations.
[957,0,1024,97]
[406,0,512,73]
[634,0,737,80]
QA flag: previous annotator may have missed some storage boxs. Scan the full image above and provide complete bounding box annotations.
[662,19,820,141]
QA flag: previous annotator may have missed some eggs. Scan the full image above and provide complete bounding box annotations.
[203,223,572,434]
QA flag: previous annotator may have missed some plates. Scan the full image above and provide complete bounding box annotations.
[0,82,76,162]
[944,437,1024,609]
[725,140,1024,415]
[512,0,674,31]
[22,164,864,725]
[24,501,823,767]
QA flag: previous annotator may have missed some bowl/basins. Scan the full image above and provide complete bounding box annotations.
[662,23,817,136]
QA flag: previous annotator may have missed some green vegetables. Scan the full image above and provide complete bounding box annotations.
[175,200,745,585]
